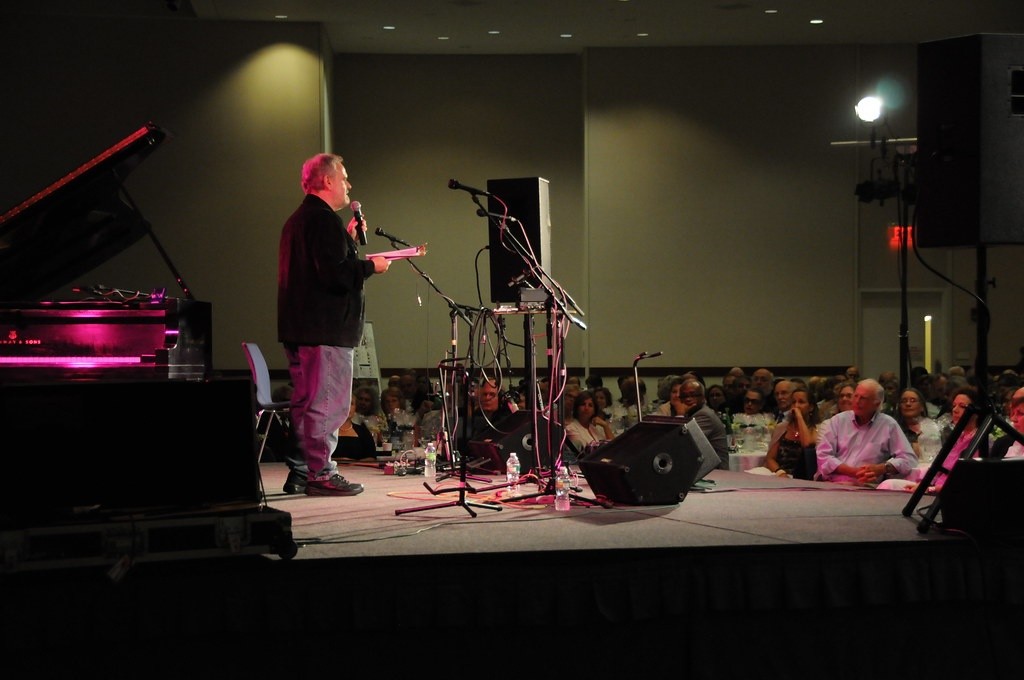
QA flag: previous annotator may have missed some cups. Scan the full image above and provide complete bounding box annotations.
[382,443,392,451]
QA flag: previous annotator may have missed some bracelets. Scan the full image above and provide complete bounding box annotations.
[775,468,781,473]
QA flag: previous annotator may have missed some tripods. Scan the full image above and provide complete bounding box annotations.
[902,245,1024,534]
[389,189,613,518]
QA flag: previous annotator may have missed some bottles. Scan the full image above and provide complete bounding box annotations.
[425,442,437,477]
[506,453,520,496]
[555,466,570,511]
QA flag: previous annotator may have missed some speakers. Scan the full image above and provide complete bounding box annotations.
[486,177,552,302]
[917,32,1024,248]
[577,414,721,506]
[941,456,1023,534]
[468,409,563,473]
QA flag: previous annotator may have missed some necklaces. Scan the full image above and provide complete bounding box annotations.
[793,422,799,438]
[339,425,352,432]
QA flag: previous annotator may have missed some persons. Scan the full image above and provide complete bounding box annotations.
[260,361,1024,490]
[277,153,390,496]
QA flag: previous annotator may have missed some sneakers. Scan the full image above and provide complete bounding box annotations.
[282,471,306,494]
[305,474,363,495]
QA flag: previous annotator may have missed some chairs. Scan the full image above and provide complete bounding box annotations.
[241,342,293,464]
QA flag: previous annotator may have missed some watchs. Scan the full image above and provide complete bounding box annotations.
[885,462,892,474]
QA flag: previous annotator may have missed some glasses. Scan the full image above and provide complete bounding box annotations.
[738,383,751,388]
[746,397,760,405]
[680,392,702,399]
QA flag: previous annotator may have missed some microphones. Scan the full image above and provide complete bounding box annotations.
[374,228,411,246]
[476,209,517,222]
[448,178,493,198]
[350,201,367,246]
